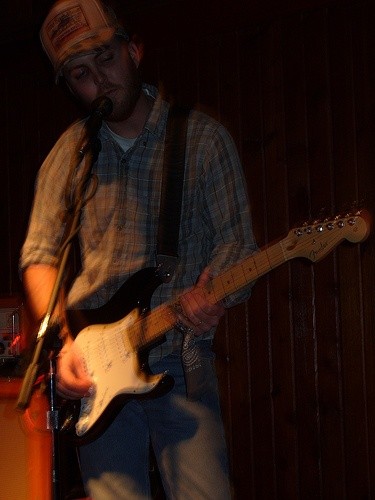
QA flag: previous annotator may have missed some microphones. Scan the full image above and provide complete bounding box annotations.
[68,97,115,175]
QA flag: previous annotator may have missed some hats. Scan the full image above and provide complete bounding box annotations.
[39,0,129,85]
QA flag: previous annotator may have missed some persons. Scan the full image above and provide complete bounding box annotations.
[22,0,260,498]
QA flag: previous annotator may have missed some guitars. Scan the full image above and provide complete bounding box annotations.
[48,210,375,446]
